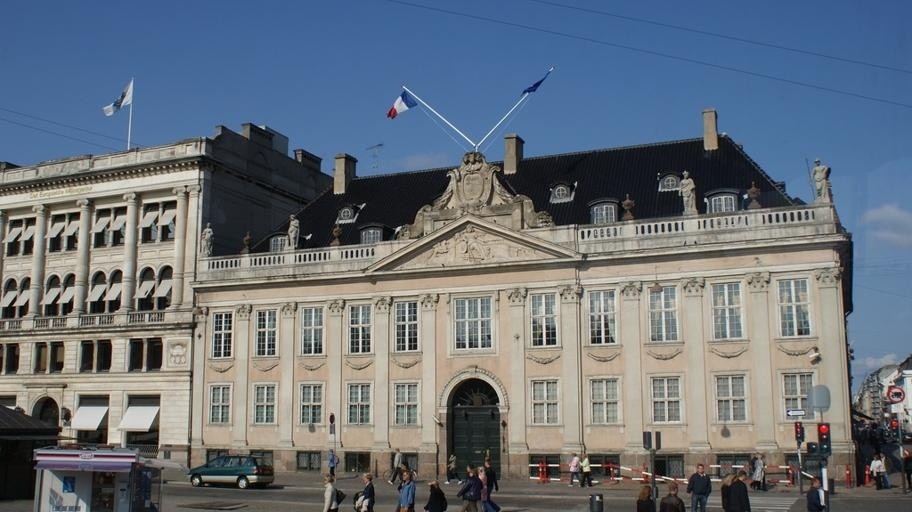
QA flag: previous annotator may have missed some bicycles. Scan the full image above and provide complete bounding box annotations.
[382,467,418,484]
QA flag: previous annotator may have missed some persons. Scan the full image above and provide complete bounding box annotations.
[637,451,767,512]
[880,453,893,490]
[904,450,912,493]
[579,454,593,487]
[807,478,828,512]
[323,448,498,512]
[870,455,885,490]
[568,453,581,487]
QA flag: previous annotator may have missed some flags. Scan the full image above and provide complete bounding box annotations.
[386,90,418,119]
[520,70,552,96]
[102,81,133,117]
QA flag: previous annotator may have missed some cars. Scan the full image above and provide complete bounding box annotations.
[184,452,276,491]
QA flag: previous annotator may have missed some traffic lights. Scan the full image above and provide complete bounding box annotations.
[807,442,817,452]
[795,421,803,442]
[817,423,832,456]
[892,420,900,437]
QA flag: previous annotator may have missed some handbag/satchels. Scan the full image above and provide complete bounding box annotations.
[336,489,346,505]
